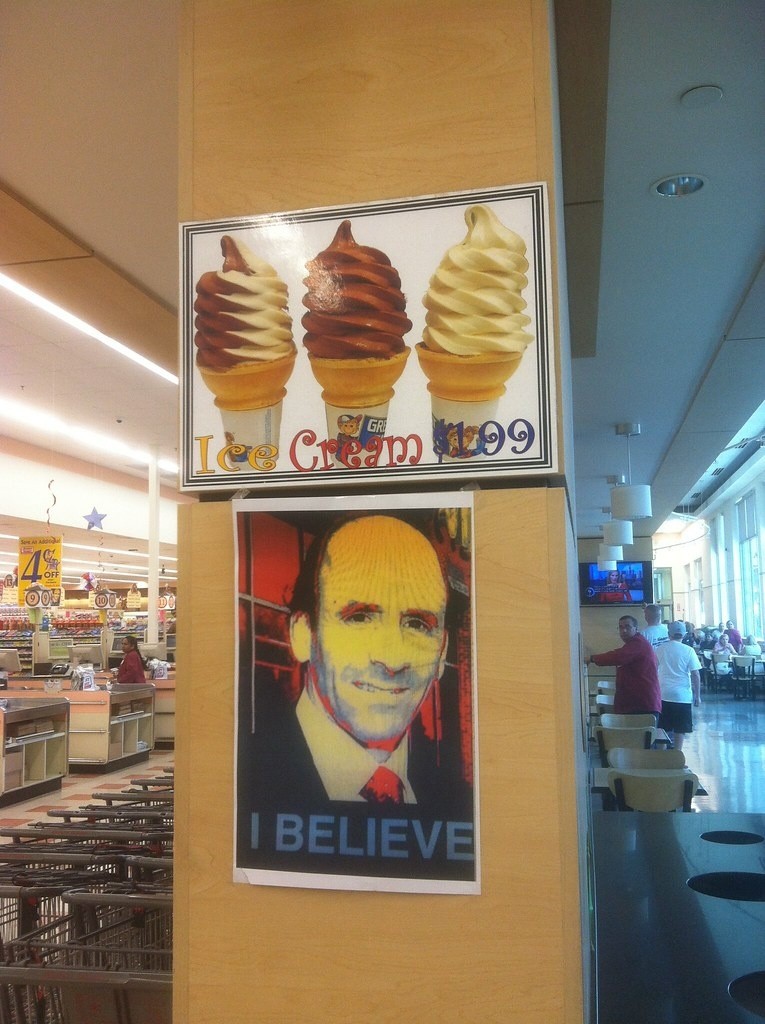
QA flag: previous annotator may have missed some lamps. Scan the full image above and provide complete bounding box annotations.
[595,422,653,571]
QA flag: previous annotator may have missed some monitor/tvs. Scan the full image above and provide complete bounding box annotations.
[0,649,21,672]
[140,642,167,660]
[578,561,653,608]
[72,643,104,664]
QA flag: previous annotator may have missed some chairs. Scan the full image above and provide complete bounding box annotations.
[701,648,758,701]
[597,680,700,812]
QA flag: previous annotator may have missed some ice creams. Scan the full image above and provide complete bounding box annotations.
[414,203,536,463]
[301,219,413,469]
[193,235,299,472]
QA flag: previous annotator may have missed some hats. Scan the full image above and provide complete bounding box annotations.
[668,621,686,638]
[706,631,714,637]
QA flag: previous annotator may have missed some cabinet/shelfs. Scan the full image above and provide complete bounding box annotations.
[0,600,177,679]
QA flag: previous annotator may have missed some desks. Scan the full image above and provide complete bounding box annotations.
[754,659,765,697]
[589,728,673,767]
[591,766,709,812]
[589,689,598,716]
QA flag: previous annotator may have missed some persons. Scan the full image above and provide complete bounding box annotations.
[50,589,61,606]
[637,602,670,651]
[600,569,632,603]
[712,634,738,693]
[679,620,726,680]
[724,620,742,654]
[110,636,149,683]
[739,636,761,656]
[584,615,662,727]
[234,511,476,883]
[653,620,702,750]
